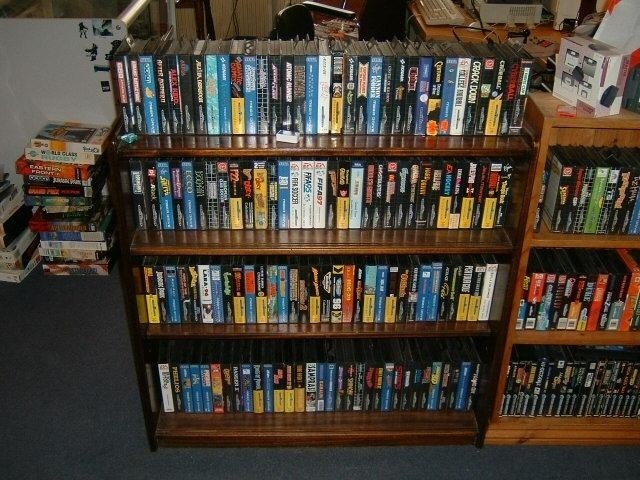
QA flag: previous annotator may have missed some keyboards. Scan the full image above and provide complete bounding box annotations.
[415,0,466,27]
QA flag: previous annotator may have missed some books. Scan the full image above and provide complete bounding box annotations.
[515,247,640,332]
[533,148,640,236]
[115,34,532,135]
[499,346,640,419]
[132,256,511,323]
[0,166,42,282]
[129,156,512,230]
[151,339,483,414]
[16,122,113,276]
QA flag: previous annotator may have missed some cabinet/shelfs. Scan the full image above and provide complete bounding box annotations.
[1,3,176,187]
[482,2,639,448]
[104,41,535,453]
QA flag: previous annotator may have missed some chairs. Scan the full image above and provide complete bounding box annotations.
[272,5,315,39]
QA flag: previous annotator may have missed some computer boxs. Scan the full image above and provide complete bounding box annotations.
[462,0,543,24]
